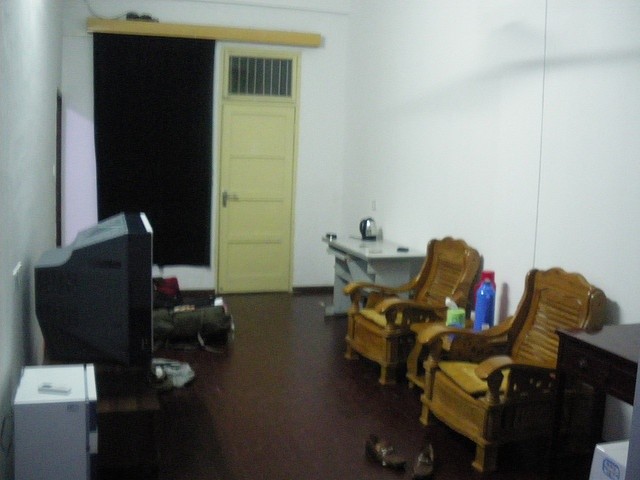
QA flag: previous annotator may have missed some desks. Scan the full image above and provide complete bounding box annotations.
[321,236,422,317]
[557,325,639,480]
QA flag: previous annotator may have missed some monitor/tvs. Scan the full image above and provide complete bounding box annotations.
[35,212,152,372]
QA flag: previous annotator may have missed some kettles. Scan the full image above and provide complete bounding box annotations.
[359,218,377,240]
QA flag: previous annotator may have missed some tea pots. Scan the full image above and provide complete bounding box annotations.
[472,269,497,307]
[474,278,496,332]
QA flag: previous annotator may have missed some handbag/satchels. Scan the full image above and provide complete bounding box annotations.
[165,306,231,354]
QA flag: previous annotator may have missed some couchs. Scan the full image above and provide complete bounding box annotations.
[417,267,606,473]
[343,236,481,384]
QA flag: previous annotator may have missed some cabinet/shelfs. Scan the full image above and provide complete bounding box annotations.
[42,349,161,480]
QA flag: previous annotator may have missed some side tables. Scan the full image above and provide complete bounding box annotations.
[406,320,504,392]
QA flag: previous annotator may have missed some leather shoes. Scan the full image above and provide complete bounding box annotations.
[365,433,406,469]
[413,442,434,477]
